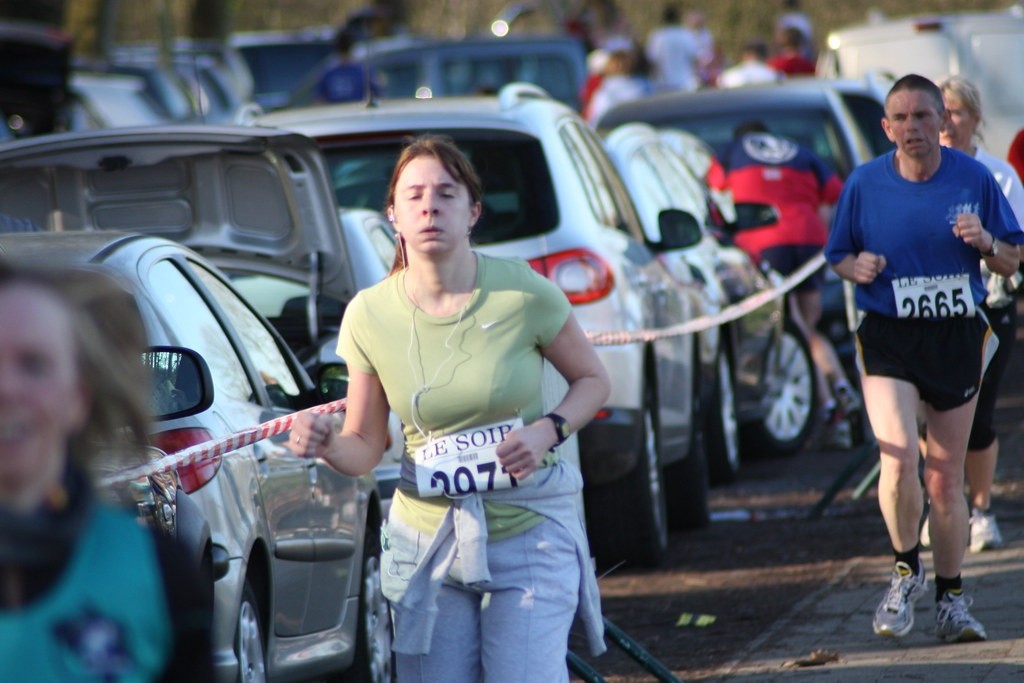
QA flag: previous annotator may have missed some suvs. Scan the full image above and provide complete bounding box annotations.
[225,81,715,570]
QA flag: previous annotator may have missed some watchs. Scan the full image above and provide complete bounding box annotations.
[545,412,571,453]
[981,235,999,257]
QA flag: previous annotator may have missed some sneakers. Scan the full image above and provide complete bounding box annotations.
[872,558,927,637]
[968,497,1002,553]
[936,588,987,641]
[919,515,933,549]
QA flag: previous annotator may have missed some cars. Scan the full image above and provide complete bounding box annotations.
[1,219,398,681]
[5,7,1024,465]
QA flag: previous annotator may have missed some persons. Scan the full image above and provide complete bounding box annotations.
[581,0,814,125]
[709,120,861,450]
[287,131,613,683]
[0,251,218,683]
[823,73,1024,642]
[919,78,1024,555]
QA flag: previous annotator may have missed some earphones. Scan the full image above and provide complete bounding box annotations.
[390,209,394,220]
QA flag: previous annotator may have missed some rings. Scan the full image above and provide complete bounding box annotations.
[297,438,300,443]
